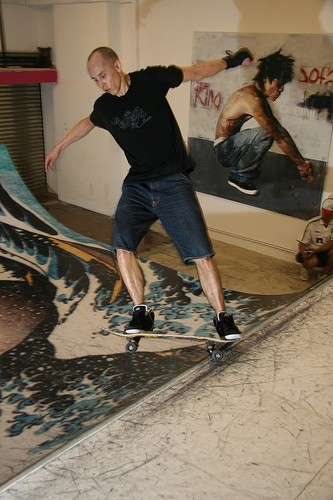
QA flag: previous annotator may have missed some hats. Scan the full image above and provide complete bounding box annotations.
[322,198,333,216]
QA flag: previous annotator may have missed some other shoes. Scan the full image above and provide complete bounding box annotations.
[228,176,257,195]
[300,267,310,280]
[317,273,327,282]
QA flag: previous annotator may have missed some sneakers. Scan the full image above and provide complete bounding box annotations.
[125,304,155,335]
[213,312,243,340]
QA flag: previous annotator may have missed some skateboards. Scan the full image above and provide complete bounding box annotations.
[98,328,243,364]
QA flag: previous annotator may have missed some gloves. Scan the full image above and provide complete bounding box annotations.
[222,47,253,69]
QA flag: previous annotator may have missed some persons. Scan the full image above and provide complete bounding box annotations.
[44,47,267,340]
[293,198,333,280]
[213,48,315,198]
